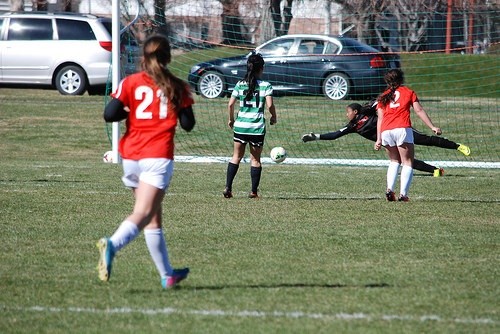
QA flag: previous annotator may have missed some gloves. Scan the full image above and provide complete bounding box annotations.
[302,132,320,143]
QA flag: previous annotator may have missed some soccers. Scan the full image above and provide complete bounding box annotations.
[103,150,113,164]
[269,146,288,164]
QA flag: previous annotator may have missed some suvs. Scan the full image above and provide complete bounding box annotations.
[0,9,142,97]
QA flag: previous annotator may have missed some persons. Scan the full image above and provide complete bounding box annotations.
[96,35,196,290]
[301,103,471,177]
[222,55,277,198]
[374,69,442,201]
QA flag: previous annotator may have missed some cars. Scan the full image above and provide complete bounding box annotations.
[188,33,404,100]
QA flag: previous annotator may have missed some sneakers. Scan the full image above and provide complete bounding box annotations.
[161,267,190,290]
[433,168,444,177]
[457,143,470,156]
[385,189,411,202]
[97,237,113,282]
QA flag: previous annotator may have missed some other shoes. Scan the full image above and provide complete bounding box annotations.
[222,187,232,198]
[248,192,257,199]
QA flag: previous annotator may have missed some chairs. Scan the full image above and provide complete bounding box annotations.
[298,45,308,54]
[313,45,324,54]
